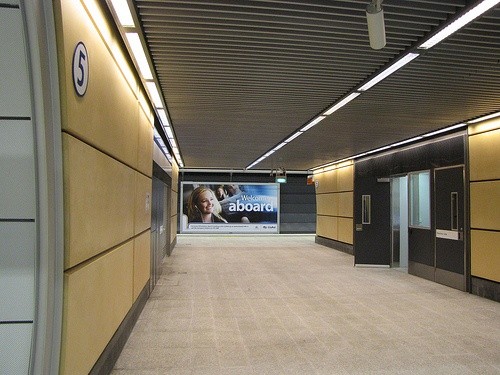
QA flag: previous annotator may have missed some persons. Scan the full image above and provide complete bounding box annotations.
[182,183,250,224]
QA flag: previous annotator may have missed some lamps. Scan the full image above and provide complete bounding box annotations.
[367,0,386,50]
